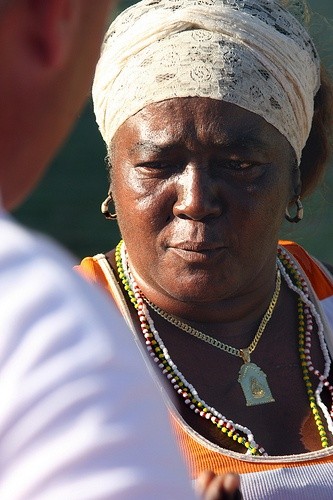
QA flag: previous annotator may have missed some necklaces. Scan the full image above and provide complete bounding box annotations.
[115,239,333,456]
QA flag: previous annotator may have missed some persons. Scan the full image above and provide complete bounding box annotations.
[0,1,197,499]
[76,0,333,500]
